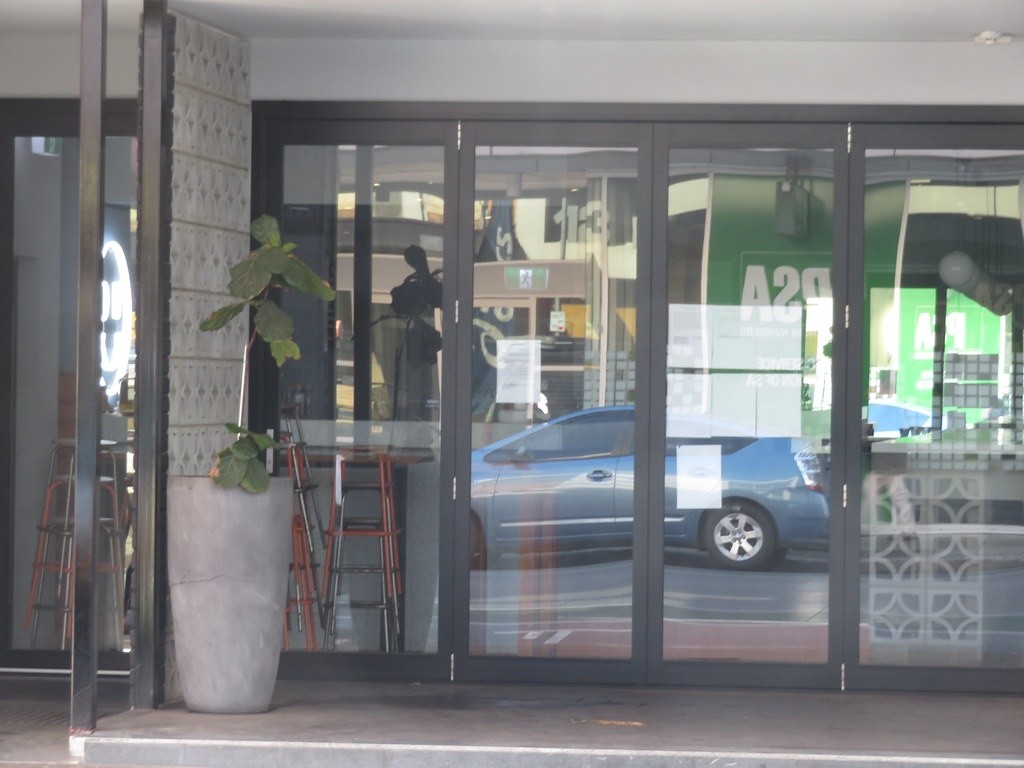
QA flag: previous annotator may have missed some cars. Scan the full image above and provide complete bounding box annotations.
[470,406,831,569]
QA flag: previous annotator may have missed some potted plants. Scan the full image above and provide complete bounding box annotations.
[166,214,336,712]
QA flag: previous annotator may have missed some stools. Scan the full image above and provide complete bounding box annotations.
[273,401,401,654]
[22,437,127,653]
